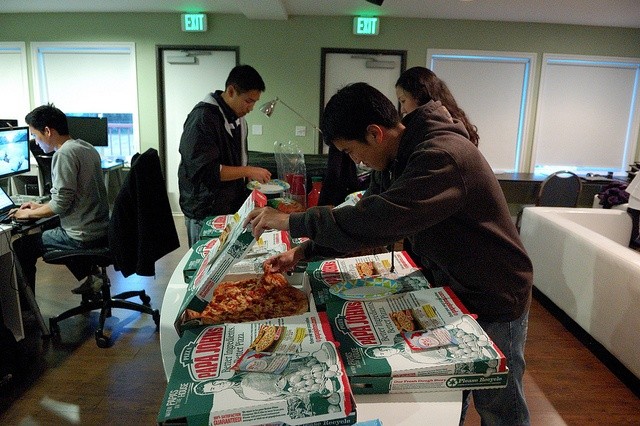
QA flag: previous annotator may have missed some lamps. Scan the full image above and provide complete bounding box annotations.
[516,171,582,233]
[41,148,162,348]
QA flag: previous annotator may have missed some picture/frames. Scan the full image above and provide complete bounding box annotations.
[0,193,60,341]
[11,162,124,198]
[159,240,463,426]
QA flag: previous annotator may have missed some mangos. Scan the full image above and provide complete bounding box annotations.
[271,196,306,215]
[248,325,278,352]
[180,263,310,324]
[354,262,377,280]
[390,309,415,335]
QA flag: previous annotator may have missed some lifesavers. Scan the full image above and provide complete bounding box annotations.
[25,184,39,196]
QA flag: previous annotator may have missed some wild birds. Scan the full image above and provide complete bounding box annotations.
[0,184,40,227]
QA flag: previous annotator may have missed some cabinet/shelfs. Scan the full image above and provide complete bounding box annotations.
[286,173,305,203]
[307,176,322,207]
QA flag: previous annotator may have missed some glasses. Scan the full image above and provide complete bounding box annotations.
[259,98,323,136]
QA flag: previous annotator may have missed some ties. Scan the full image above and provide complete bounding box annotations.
[67,116,108,147]
[0,127,31,179]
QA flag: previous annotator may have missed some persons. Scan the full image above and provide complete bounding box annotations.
[194,357,312,402]
[178,64,273,250]
[242,80,534,425]
[7,103,110,294]
[364,325,460,365]
[394,65,481,149]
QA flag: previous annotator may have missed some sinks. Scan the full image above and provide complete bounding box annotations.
[520,207,640,399]
